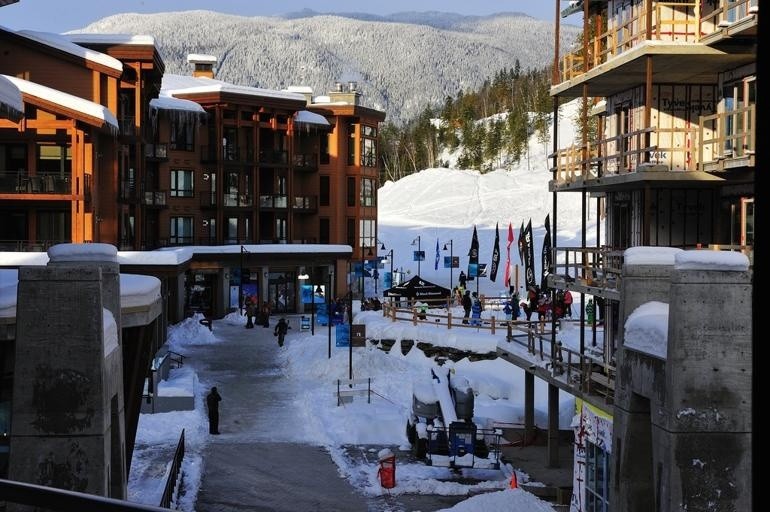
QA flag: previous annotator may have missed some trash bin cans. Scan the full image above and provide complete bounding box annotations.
[379,454,395,488]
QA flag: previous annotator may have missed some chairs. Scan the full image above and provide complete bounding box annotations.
[30,175,55,193]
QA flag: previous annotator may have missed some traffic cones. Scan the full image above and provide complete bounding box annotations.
[511,470,518,488]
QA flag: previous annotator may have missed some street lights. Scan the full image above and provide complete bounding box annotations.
[316,272,331,359]
[361,242,373,310]
[380,249,394,306]
[411,235,421,277]
[374,239,385,294]
[443,240,453,289]
[298,269,315,335]
[238,245,251,316]
[335,289,354,388]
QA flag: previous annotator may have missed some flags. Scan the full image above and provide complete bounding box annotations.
[434,238,441,270]
[516,223,525,266]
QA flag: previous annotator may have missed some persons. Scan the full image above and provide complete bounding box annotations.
[536,294,549,319]
[509,294,520,320]
[523,304,532,321]
[359,296,383,313]
[470,298,482,327]
[244,303,255,329]
[543,287,577,320]
[458,270,467,289]
[207,386,223,435]
[273,318,288,346]
[585,298,594,326]
[460,290,472,324]
[419,309,426,320]
[333,297,344,323]
